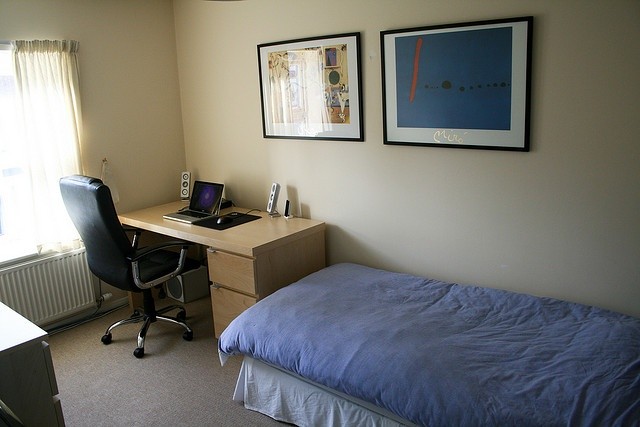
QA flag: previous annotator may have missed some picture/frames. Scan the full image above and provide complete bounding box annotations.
[377,17,533,148]
[254,32,364,142]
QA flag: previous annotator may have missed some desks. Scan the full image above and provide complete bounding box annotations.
[107,191,325,367]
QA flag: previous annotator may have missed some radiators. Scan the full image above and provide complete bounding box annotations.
[0,247,97,326]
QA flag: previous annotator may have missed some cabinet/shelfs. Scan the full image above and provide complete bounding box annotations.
[0,301,69,427]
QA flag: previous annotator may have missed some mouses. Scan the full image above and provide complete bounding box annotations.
[216,217,232,224]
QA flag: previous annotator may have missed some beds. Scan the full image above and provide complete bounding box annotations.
[213,260,635,426]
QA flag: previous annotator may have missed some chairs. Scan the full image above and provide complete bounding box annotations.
[60,174,204,358]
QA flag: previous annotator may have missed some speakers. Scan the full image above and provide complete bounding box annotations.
[266,183,281,218]
[181,172,191,201]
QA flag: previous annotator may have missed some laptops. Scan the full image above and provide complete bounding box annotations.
[162,181,225,223]
